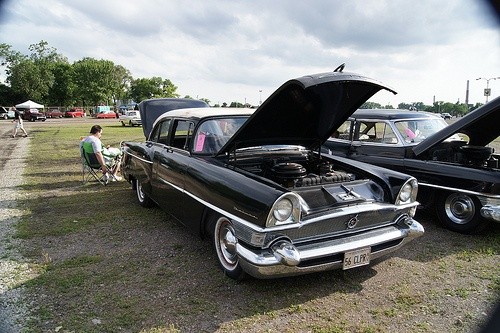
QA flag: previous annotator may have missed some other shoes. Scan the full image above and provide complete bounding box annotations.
[10,136,15,138]
[104,173,109,180]
[109,174,123,181]
[21,134,28,137]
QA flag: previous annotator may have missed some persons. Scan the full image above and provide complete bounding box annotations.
[10,105,28,138]
[81,125,122,185]
[83,108,127,119]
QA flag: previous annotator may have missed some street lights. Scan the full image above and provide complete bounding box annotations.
[475,76,500,103]
[258,90,262,105]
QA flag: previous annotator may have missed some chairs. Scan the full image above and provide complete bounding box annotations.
[80,146,120,187]
[358,134,407,144]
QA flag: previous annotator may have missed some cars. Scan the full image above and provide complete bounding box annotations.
[96,111,117,119]
[120,111,143,128]
[442,113,452,118]
[45,109,63,119]
[66,109,85,118]
[118,63,425,281]
[0,106,47,122]
[318,95,500,232]
